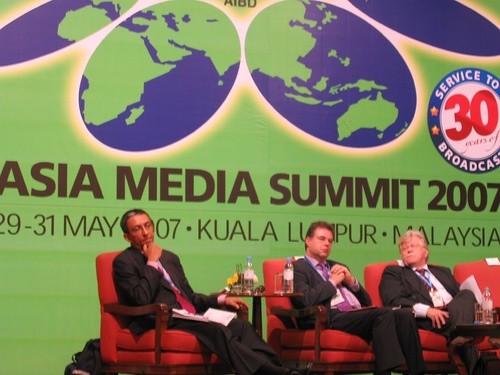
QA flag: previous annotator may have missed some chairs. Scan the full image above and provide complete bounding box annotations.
[262,258,377,375]
[96,252,249,375]
[365,261,500,375]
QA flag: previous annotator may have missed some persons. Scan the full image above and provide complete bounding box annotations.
[379,231,479,375]
[113,210,302,375]
[283,221,427,375]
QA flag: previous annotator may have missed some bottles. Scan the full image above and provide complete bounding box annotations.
[482,287,492,324]
[243,256,254,293]
[284,257,293,293]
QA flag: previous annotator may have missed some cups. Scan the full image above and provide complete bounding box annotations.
[274,273,284,293]
[231,272,242,293]
[475,303,483,324]
[488,309,497,323]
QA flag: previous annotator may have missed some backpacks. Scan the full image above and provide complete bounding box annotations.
[65,339,117,375]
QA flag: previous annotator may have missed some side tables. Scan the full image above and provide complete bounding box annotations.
[210,293,303,339]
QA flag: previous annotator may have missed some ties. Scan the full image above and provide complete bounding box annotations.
[317,263,354,312]
[159,267,196,314]
[415,269,437,292]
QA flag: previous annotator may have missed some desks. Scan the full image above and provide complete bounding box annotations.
[456,323,500,375]
